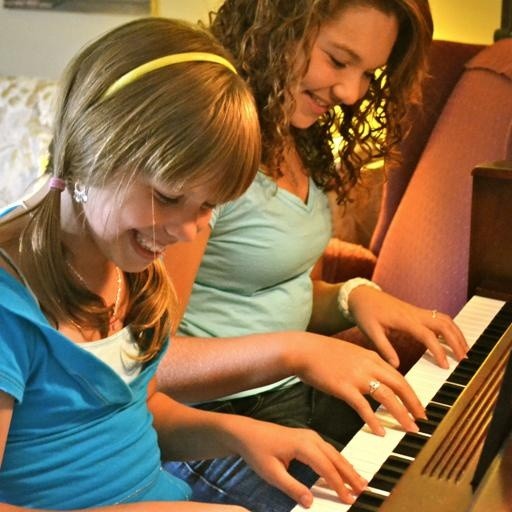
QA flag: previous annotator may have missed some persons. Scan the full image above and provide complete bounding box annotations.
[0,13,372,511]
[153,2,468,454]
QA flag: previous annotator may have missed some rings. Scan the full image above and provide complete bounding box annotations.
[368,379,382,399]
[430,306,439,318]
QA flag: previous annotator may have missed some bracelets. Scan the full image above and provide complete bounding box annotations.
[335,275,383,325]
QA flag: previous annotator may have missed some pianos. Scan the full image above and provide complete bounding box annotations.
[288,163,511,511]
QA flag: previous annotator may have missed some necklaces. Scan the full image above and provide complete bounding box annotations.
[17,197,127,339]
